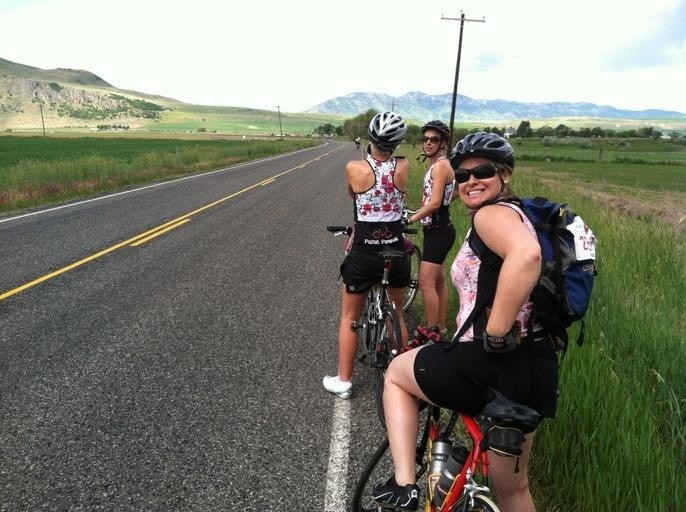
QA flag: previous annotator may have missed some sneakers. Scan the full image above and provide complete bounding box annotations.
[372,474,419,512]
[414,328,448,337]
[322,376,353,399]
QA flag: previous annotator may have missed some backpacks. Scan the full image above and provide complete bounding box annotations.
[468,196,599,361]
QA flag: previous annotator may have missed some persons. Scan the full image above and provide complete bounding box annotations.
[321,111,409,400]
[370,130,559,512]
[353,136,360,147]
[405,120,455,334]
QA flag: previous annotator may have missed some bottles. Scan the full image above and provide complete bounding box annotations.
[433,445,470,508]
[431,431,451,493]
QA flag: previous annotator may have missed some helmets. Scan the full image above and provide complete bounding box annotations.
[421,120,451,142]
[449,131,515,170]
[368,112,408,151]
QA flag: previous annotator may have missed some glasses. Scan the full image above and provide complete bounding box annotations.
[422,136,439,144]
[455,163,498,183]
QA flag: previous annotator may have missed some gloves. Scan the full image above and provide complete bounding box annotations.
[483,320,522,354]
